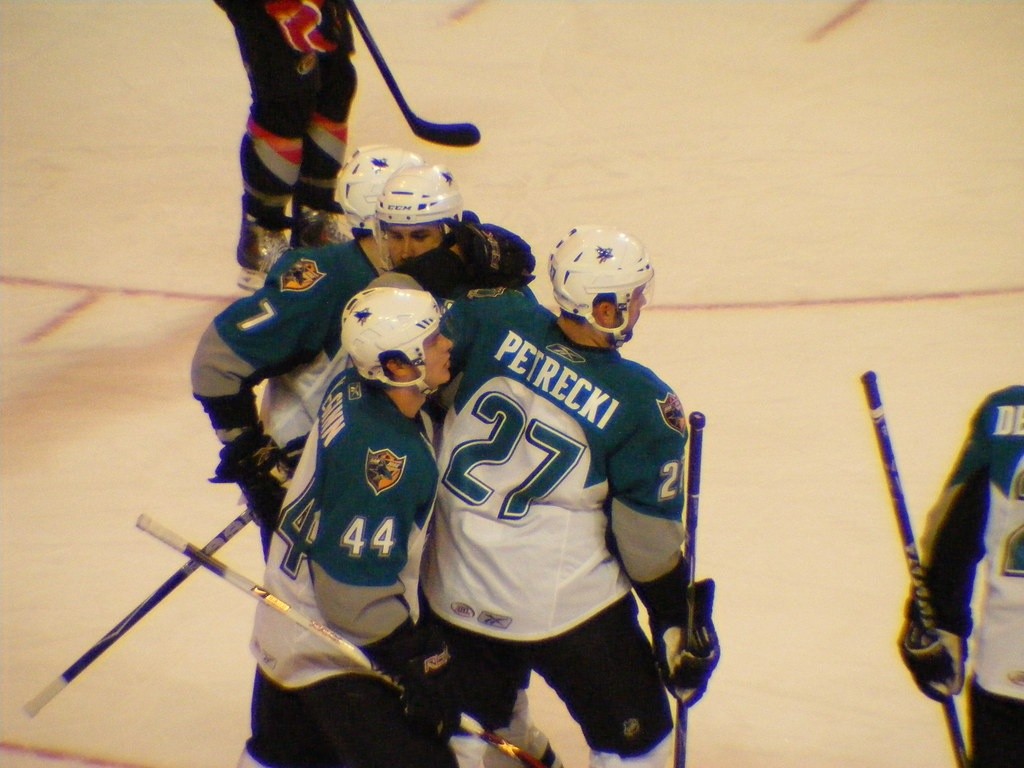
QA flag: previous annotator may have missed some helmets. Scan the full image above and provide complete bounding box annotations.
[548,225,654,316]
[334,146,422,220]
[373,163,462,229]
[340,286,442,379]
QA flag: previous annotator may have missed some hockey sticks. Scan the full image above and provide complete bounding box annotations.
[133,509,549,767]
[23,509,254,722]
[858,368,973,768]
[673,407,707,768]
[344,0,483,149]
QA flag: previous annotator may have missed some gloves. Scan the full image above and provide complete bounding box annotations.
[445,215,531,285]
[649,577,720,706]
[386,622,474,745]
[898,593,973,700]
[210,421,297,529]
[265,0,342,55]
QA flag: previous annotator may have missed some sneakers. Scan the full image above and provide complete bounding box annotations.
[289,197,353,249]
[236,209,293,291]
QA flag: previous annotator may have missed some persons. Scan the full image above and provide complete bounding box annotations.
[372,162,466,272]
[898,383,1024,768]
[419,227,720,768]
[237,268,484,768]
[212,1,359,299]
[190,144,426,569]
